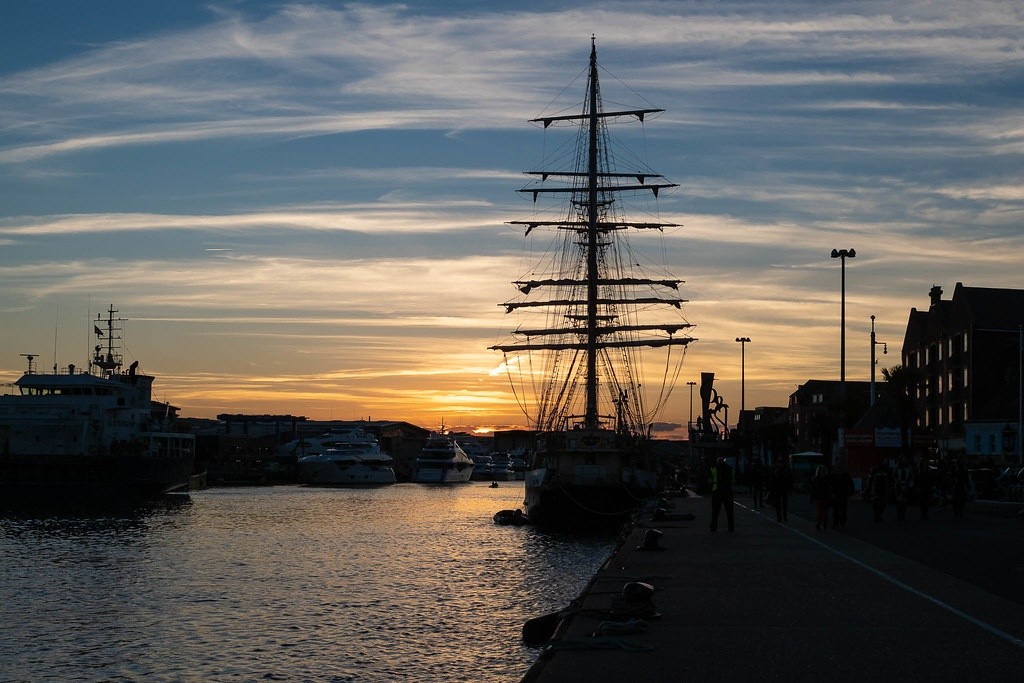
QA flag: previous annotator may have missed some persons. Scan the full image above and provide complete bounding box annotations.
[697,415,704,429]
[707,456,737,532]
[746,453,1004,536]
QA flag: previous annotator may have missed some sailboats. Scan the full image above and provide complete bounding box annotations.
[484,32,699,538]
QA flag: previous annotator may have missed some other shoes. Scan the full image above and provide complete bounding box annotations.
[841,524,846,529]
[823,523,827,529]
[782,512,787,521]
[709,528,716,532]
[778,517,781,522]
[726,528,733,532]
[831,525,839,528]
[816,523,820,530]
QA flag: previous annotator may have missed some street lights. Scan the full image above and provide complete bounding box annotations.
[734,337,753,411]
[687,382,696,427]
[830,247,858,403]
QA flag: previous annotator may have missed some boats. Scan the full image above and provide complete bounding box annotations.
[411,416,476,483]
[298,428,398,487]
[1,301,196,505]
[473,451,517,483]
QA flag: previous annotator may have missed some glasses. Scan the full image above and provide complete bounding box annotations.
[819,469,825,471]
